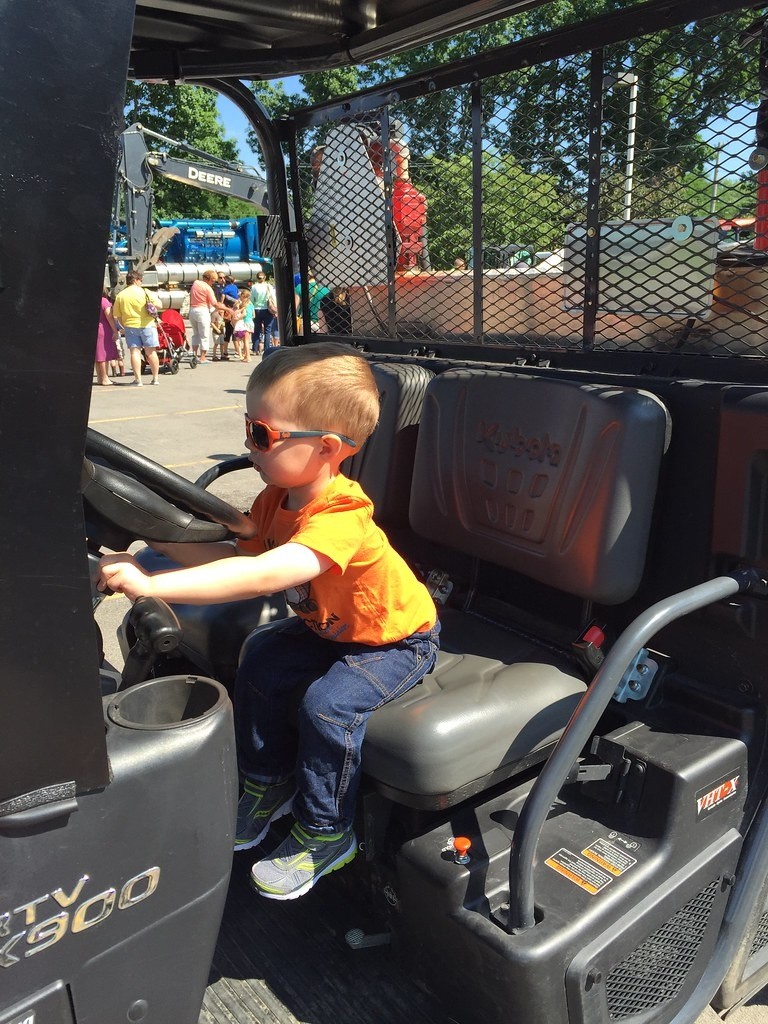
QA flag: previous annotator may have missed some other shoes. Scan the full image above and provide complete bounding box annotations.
[192,344,280,364]
[130,381,144,387]
[151,380,160,385]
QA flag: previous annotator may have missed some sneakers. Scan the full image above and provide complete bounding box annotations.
[249,822,358,901]
[233,777,300,851]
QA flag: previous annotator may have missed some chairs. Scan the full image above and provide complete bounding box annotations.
[310,369,674,814]
[128,360,436,672]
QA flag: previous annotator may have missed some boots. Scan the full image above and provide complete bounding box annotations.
[119,365,126,377]
[111,366,118,377]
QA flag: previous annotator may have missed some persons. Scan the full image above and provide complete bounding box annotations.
[96,270,352,387]
[92,343,442,901]
[455,258,465,270]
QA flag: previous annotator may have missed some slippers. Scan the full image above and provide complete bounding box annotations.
[97,379,120,386]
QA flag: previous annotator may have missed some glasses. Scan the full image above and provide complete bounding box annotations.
[218,277,226,280]
[244,412,357,454]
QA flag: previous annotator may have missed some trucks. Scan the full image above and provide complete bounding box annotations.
[0,0,768,1024]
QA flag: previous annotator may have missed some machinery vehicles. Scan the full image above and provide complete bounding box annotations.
[112,122,300,275]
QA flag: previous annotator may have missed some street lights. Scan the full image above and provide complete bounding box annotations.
[603,72,637,224]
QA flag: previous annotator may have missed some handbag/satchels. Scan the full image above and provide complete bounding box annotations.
[142,288,158,318]
[267,285,276,315]
[179,293,191,319]
